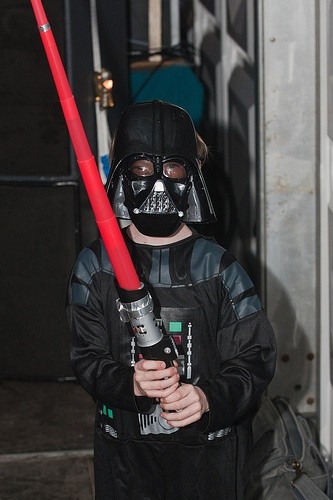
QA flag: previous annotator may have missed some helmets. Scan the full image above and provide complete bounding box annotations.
[104,100,217,239]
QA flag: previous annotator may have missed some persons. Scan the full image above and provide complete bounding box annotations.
[64,98,277,500]
